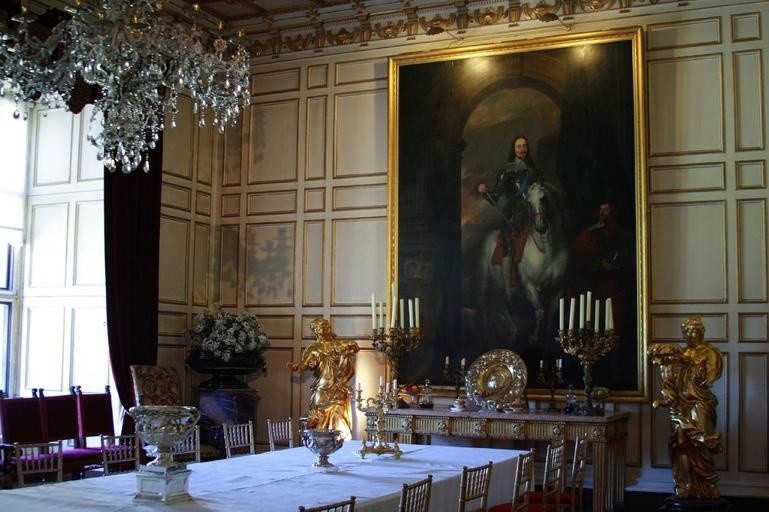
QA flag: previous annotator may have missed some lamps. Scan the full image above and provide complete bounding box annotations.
[0,0,253,174]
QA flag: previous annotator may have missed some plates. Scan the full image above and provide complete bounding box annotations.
[463,348,528,410]
[450,407,467,412]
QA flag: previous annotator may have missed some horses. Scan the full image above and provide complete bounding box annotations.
[474,172,574,347]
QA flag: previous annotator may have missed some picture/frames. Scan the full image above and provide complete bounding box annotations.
[387,25,651,403]
[0,300,12,398]
[0,238,14,291]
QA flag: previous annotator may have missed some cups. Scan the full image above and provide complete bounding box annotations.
[454,397,465,408]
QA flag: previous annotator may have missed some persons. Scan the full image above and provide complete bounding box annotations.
[287,316,360,443]
[572,203,631,309]
[646,315,725,500]
[479,136,556,301]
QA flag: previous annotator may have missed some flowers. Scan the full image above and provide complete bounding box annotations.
[182,307,269,362]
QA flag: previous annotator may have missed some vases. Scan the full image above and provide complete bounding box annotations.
[188,345,264,389]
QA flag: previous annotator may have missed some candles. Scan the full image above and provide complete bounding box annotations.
[358,382,361,389]
[446,356,449,364]
[372,292,420,329]
[379,376,398,393]
[559,359,562,368]
[560,291,614,333]
[461,358,466,365]
[540,360,543,368]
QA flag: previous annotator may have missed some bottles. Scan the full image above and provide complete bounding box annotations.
[421,379,434,405]
[565,384,577,409]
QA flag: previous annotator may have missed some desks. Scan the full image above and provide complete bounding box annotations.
[365,407,628,512]
[194,386,261,427]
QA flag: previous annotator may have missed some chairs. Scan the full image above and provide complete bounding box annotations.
[130,364,221,461]
[267,417,294,451]
[222,421,255,458]
[14,440,62,486]
[0,386,136,487]
[299,432,588,512]
[170,426,200,462]
[101,433,140,476]
